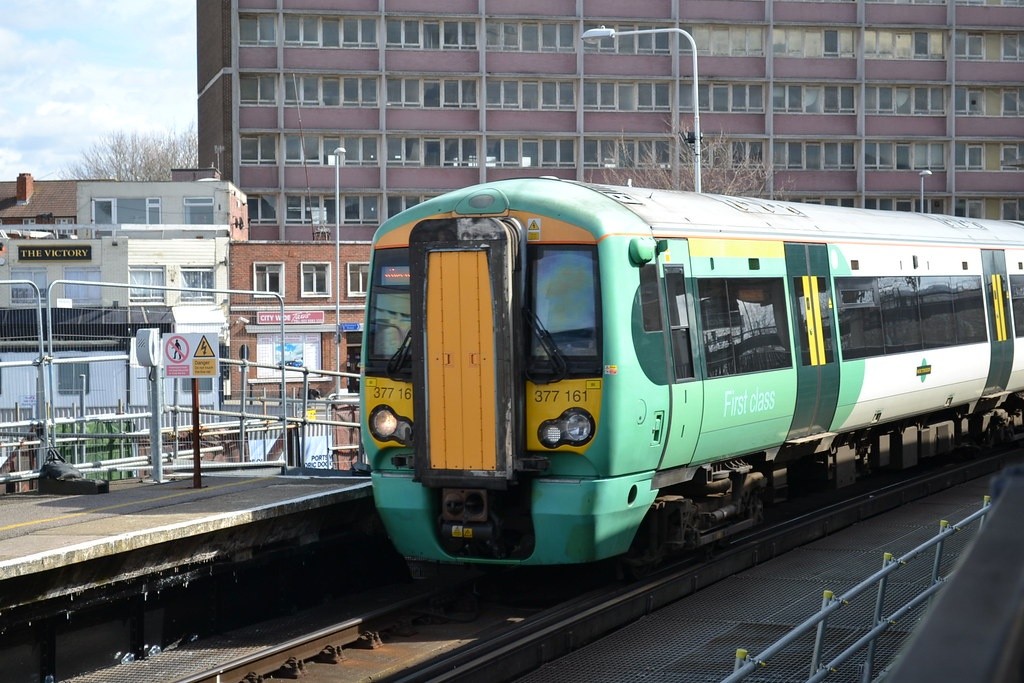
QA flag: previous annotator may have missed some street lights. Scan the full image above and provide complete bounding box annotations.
[581,24,703,193]
[332,147,347,399]
[918,169,932,214]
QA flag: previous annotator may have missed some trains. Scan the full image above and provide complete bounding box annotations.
[356,173,1024,584]
